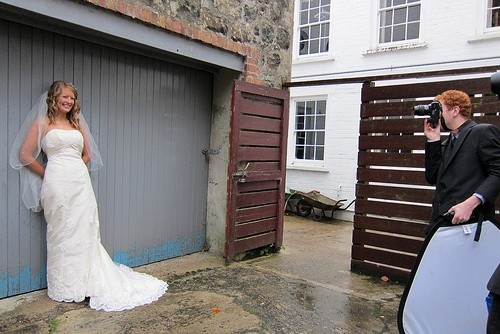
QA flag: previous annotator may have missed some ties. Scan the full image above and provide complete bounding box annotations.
[443,136,457,166]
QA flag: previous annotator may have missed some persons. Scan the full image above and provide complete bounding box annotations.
[19,80,114,302]
[422,89,500,237]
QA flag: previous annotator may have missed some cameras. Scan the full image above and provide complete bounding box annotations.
[413,102,443,124]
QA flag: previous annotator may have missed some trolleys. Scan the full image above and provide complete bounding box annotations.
[289,189,355,221]
